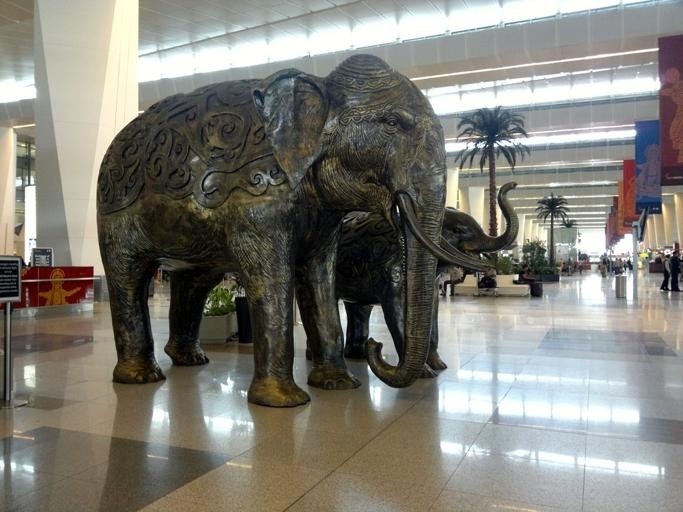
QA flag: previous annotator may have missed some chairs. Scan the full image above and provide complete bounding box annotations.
[497,275,532,297]
[453,274,478,296]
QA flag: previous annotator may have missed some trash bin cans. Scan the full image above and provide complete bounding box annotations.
[600,264,607,277]
[615,275,627,298]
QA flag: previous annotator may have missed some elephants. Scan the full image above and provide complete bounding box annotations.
[306,182,519,378]
[98,55,496,409]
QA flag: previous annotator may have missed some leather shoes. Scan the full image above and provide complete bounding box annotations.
[661,287,679,291]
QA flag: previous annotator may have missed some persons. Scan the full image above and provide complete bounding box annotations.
[658,249,683,291]
[596,249,632,277]
[436,262,538,298]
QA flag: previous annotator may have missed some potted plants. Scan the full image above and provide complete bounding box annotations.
[198,272,246,344]
